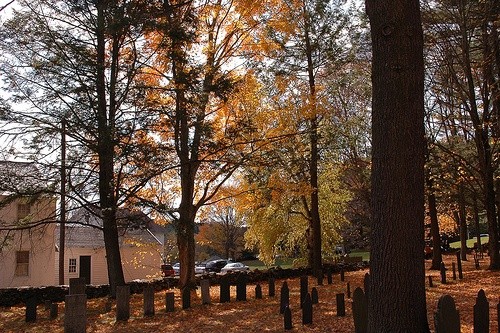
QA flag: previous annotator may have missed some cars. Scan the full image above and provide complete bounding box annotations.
[334,245,344,253]
[221,262,250,274]
[195,263,205,275]
[161,264,175,278]
[173,263,180,277]
[424,238,434,260]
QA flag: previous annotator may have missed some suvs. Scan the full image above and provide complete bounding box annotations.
[206,259,232,273]
